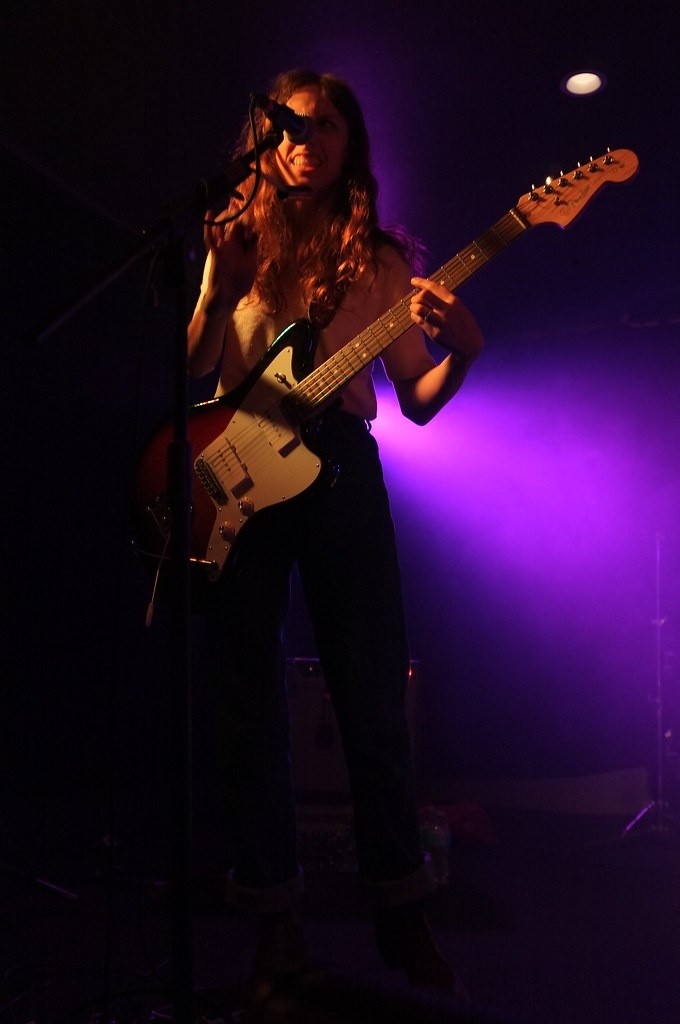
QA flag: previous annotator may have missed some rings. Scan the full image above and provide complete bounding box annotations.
[427,310,433,318]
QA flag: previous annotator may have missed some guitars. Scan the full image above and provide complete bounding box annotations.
[129,140,641,605]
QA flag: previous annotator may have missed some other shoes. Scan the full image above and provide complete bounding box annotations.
[377,899,456,994]
[246,913,325,1024]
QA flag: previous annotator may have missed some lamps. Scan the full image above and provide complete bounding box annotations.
[557,46,608,99]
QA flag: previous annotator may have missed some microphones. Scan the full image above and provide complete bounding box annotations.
[247,86,316,145]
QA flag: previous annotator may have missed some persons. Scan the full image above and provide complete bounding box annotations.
[176,70,483,1023]
